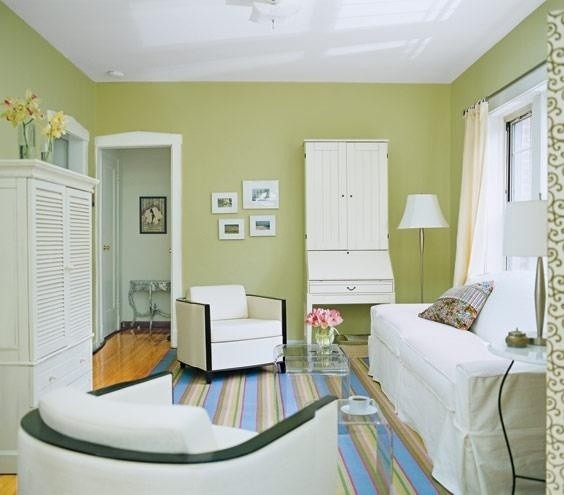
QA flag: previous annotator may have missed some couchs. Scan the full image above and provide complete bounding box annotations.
[369,265,547,495]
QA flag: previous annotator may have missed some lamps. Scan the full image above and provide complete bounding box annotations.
[248,0,300,30]
[398,191,449,302]
[497,199,548,346]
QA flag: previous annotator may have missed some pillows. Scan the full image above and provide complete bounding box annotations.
[416,281,494,329]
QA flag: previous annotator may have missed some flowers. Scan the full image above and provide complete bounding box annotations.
[0,85,44,126]
[40,113,68,136]
[306,304,341,328]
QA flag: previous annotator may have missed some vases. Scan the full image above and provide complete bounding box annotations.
[38,128,52,160]
[14,124,30,157]
[315,327,333,349]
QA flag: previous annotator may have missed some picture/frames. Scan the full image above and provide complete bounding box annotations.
[138,195,167,235]
[206,178,281,240]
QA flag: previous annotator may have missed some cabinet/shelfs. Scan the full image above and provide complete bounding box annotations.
[0,158,100,364]
[0,334,95,475]
[304,137,393,340]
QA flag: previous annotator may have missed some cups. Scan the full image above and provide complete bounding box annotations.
[347,396,377,413]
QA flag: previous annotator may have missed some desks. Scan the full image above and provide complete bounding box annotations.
[127,280,171,336]
[487,334,547,495]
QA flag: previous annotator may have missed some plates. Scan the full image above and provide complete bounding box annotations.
[342,405,376,417]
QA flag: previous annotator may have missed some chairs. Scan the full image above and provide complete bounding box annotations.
[17,373,340,495]
[172,284,287,385]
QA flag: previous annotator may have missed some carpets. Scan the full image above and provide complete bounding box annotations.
[140,346,438,495]
